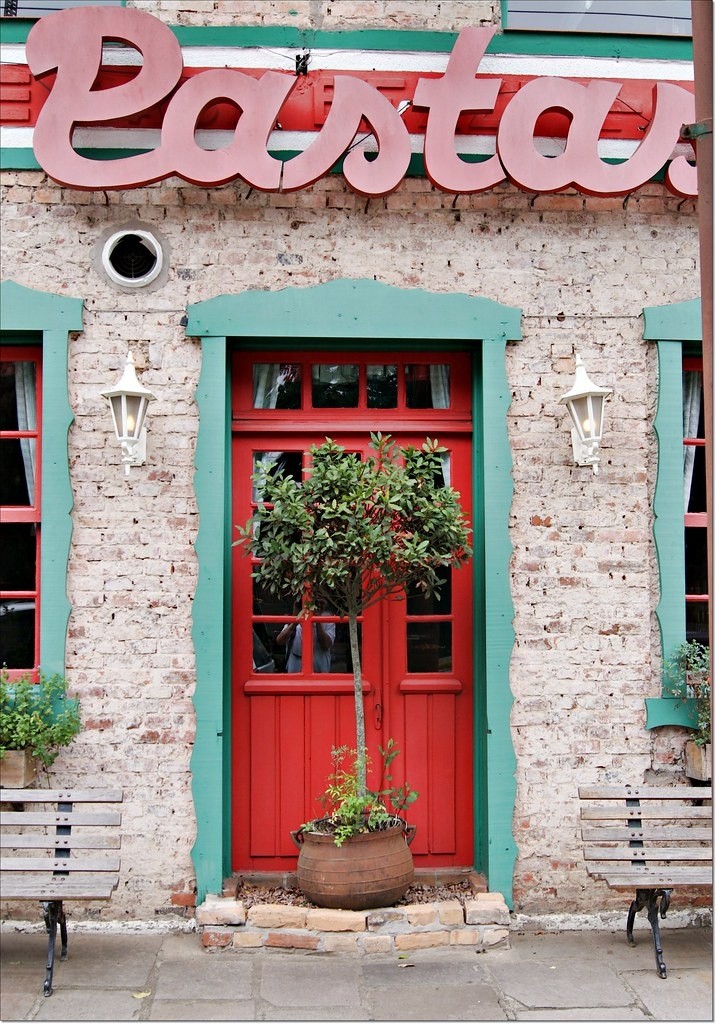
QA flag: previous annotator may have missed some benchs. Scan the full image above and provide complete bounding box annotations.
[0,790,125,997]
[577,788,712,977]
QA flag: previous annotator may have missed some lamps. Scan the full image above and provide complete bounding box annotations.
[100,361,156,476]
[561,363,615,477]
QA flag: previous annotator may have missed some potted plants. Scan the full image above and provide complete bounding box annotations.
[663,638,714,781]
[0,661,81,787]
[231,427,472,906]
